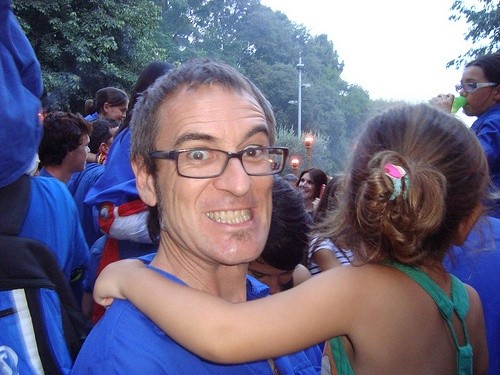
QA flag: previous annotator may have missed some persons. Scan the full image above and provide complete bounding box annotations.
[428,53,500,217]
[19,112,93,287]
[93,104,491,375]
[246,167,358,295]
[68,62,175,327]
[70,59,322,375]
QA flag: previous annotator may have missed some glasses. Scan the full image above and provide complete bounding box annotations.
[151,147,289,178]
[455,82,498,93]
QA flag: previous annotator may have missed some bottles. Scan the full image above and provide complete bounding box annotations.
[448,96,466,114]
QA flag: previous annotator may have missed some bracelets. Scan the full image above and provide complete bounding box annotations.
[95,152,105,165]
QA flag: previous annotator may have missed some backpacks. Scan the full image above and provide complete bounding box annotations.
[0,174,89,374]
